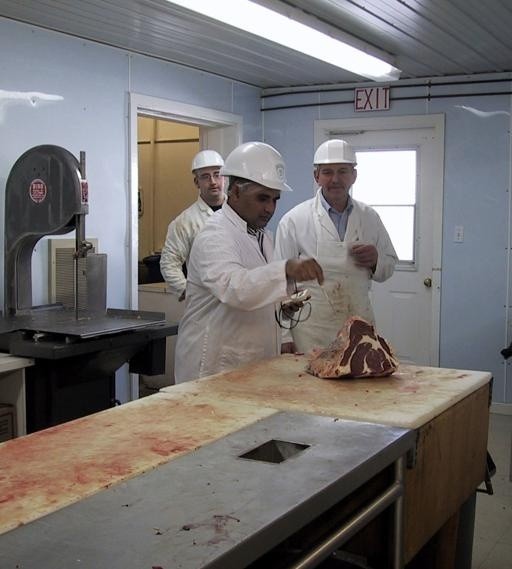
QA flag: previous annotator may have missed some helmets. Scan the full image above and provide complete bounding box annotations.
[191,149,223,175]
[219,141,294,192]
[313,139,357,164]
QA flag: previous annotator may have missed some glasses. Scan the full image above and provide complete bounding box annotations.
[196,172,221,182]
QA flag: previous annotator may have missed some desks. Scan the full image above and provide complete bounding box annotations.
[2,300,498,569]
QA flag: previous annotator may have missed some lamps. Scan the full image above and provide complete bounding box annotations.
[166,0,403,88]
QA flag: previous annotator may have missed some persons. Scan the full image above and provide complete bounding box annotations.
[173,141,325,384]
[272,136,398,354]
[158,149,230,298]
